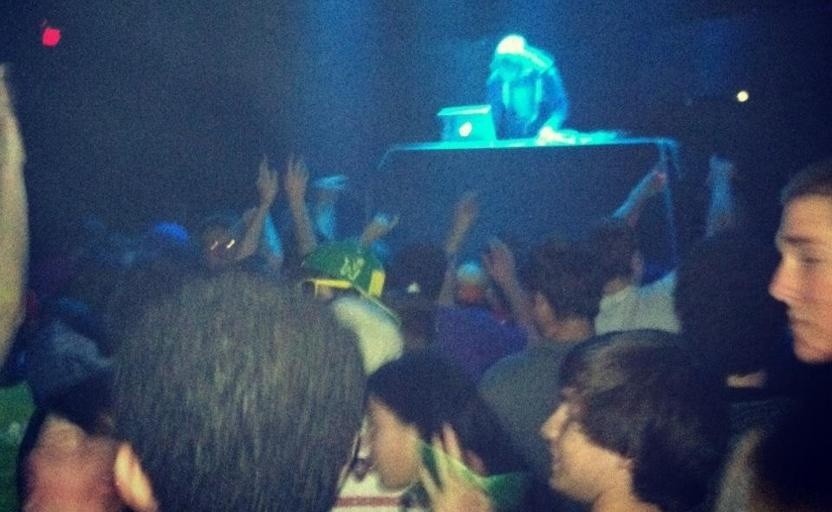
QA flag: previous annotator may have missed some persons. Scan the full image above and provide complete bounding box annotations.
[1,149,832,511]
[484,33,575,145]
[0,66,43,368]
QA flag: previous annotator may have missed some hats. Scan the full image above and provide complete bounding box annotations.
[299,240,402,334]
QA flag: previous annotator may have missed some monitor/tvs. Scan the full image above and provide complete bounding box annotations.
[437,106,497,140]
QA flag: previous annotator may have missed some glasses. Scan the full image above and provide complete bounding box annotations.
[296,279,353,301]
